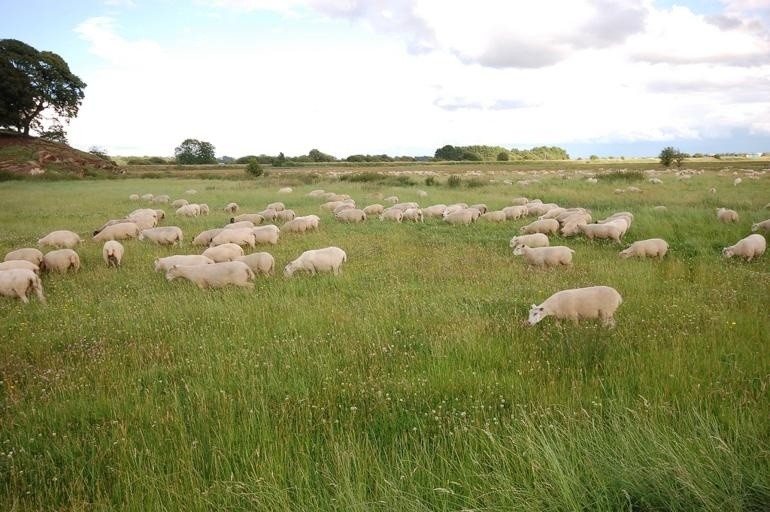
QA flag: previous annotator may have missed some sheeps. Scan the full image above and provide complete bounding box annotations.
[709,187,716,195]
[715,206,739,223]
[751,219,770,234]
[722,235,766,263]
[0,228,80,307]
[92,187,635,293]
[618,238,669,262]
[527,283,623,328]
